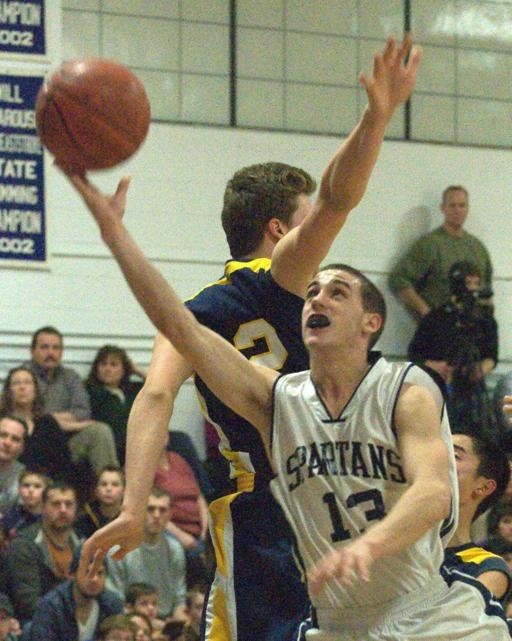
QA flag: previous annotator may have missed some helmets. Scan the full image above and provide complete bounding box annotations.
[449,261,481,295]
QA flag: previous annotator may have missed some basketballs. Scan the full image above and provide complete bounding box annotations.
[34,58,151,173]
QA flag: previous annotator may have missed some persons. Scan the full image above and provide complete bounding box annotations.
[386,185,493,317]
[0,328,210,641]
[77,36,423,641]
[48,158,512,641]
[406,259,498,434]
[421,342,512,621]
